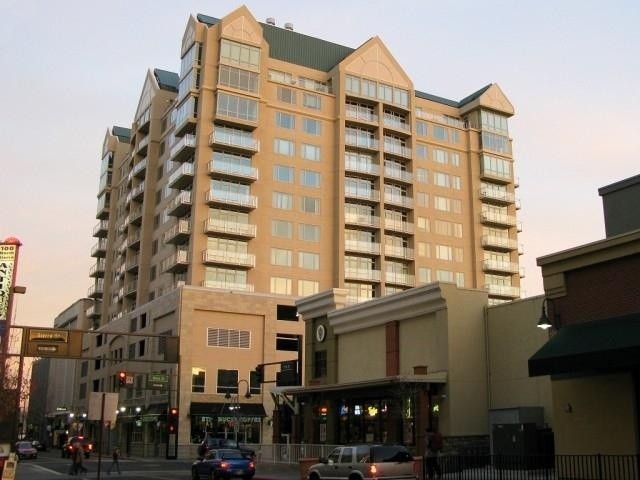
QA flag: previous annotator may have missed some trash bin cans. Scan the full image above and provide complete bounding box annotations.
[492,423,554,469]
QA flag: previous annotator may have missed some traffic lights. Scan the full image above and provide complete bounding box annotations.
[170,408,177,433]
[255,365,263,385]
[119,372,124,388]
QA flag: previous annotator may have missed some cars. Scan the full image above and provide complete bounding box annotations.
[61,436,93,459]
[307,444,421,480]
[192,438,257,480]
[15,438,45,462]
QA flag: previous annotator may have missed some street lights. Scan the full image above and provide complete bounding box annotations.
[115,407,140,457]
[70,410,86,435]
[225,380,252,448]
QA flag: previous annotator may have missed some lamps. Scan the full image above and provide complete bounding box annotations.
[536,297,561,332]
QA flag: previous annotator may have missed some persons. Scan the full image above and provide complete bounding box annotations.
[194,435,201,443]
[67,435,87,474]
[424,427,442,479]
[107,444,122,474]
